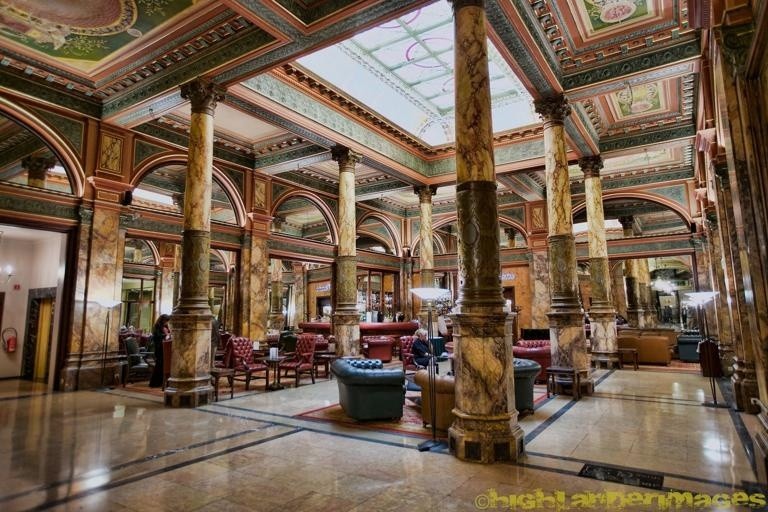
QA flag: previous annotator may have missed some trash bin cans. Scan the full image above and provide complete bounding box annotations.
[699,339,722,377]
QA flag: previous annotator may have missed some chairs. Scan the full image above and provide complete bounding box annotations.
[120,320,714,431]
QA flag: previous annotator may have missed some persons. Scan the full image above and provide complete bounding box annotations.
[412,328,437,369]
[211,318,220,368]
[148,314,171,387]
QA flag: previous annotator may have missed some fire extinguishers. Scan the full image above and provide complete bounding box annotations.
[2,328,17,353]
